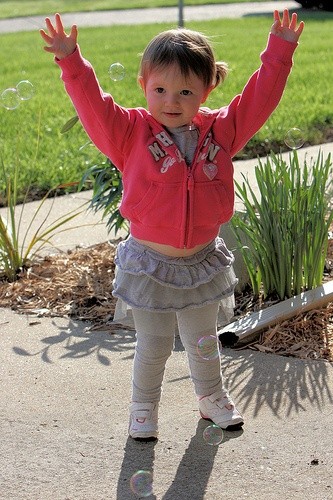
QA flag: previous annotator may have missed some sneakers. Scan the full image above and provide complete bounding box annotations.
[197,389,245,431]
[129,399,161,441]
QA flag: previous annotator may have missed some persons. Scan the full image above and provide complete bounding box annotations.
[39,7,306,444]
